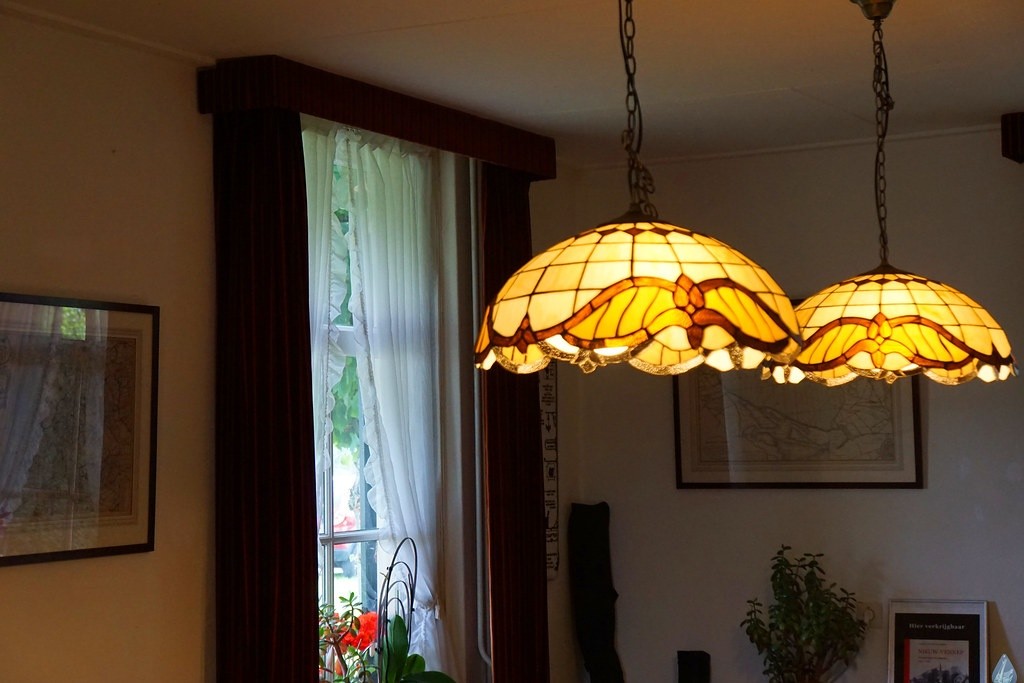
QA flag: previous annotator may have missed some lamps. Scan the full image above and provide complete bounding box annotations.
[471,1,806,376]
[760,0,1019,387]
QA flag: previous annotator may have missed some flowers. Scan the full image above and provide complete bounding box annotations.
[318,593,387,683]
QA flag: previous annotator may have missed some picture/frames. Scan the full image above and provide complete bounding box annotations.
[674,298,925,488]
[0,289,158,567]
